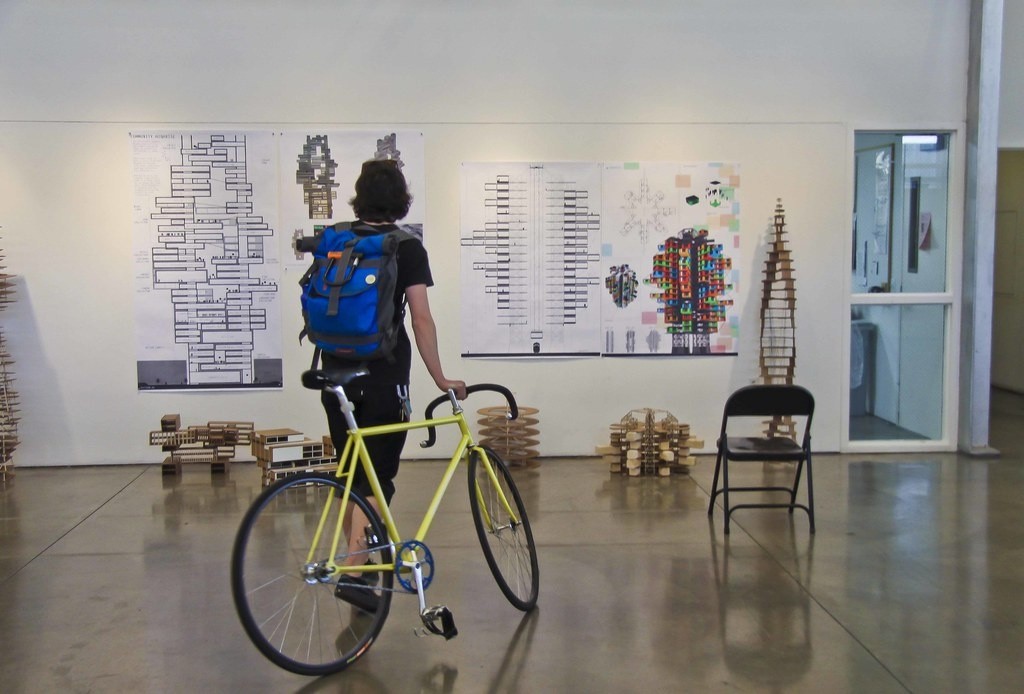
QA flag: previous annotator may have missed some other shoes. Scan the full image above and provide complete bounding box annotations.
[363,559,379,586]
[334,574,379,614]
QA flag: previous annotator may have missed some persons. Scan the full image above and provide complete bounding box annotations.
[295,160,467,613]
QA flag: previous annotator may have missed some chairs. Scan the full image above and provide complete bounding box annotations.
[708,383,816,537]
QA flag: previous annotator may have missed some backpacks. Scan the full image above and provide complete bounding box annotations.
[297,222,419,360]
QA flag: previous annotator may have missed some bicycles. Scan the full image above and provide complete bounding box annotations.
[230,366,540,677]
